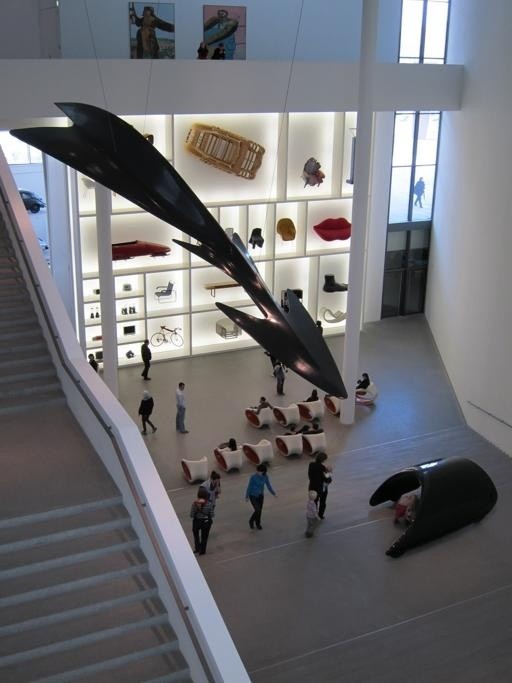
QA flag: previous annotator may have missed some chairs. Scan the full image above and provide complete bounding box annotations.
[179,381,379,484]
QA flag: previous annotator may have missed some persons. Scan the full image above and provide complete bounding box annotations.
[284,424,302,435]
[244,464,276,531]
[304,387,318,401]
[141,338,152,380]
[252,396,273,415]
[264,350,281,376]
[393,493,418,524]
[273,361,288,395]
[197,41,209,58]
[174,381,190,433]
[217,438,238,452]
[356,372,370,394]
[304,490,321,537]
[298,422,324,433]
[414,176,425,207]
[88,353,99,371]
[210,42,226,59]
[139,389,157,433]
[200,470,221,510]
[307,450,333,518]
[314,318,324,334]
[190,489,215,556]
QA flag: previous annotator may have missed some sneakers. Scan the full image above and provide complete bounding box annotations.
[152,427,157,433]
[141,373,152,381]
[180,429,190,435]
[305,531,313,539]
[317,514,326,519]
[249,520,263,530]
[141,431,147,435]
[193,548,207,556]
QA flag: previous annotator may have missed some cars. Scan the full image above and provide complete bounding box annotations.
[18,187,46,213]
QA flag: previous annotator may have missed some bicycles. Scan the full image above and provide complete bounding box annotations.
[151,326,185,347]
